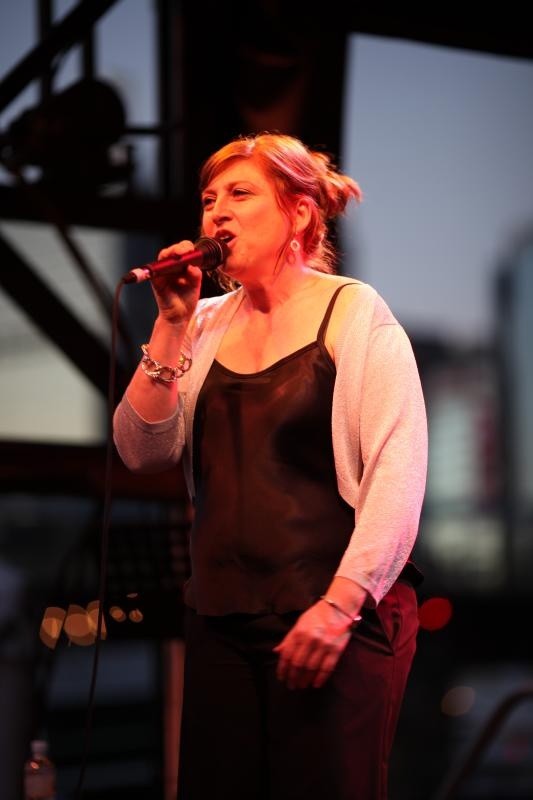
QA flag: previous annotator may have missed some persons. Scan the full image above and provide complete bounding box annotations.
[110,135,428,800]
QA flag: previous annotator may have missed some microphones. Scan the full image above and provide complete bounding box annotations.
[125,238,229,286]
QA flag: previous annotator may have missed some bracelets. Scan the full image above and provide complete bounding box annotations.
[321,596,362,624]
[139,343,192,381]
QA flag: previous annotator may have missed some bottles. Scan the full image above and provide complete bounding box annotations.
[24,742,55,800]
